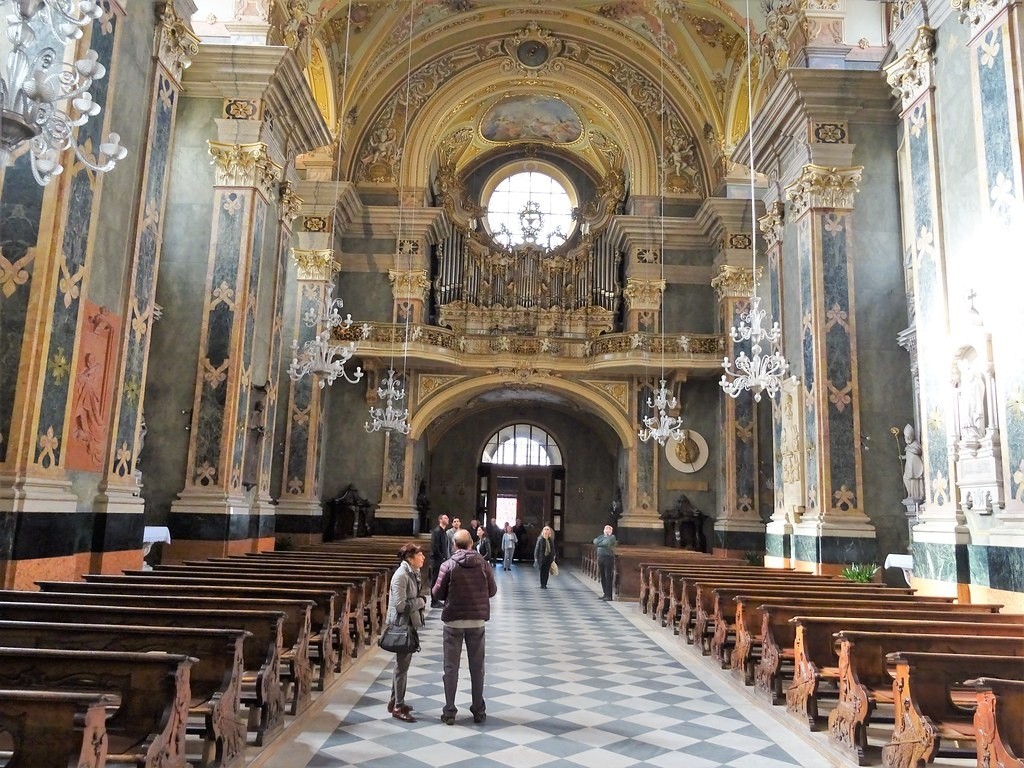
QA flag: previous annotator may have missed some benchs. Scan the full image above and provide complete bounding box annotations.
[638,562,1024,768]
[0,532,434,768]
[579,543,750,603]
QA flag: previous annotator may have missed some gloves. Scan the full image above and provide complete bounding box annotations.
[420,596,426,603]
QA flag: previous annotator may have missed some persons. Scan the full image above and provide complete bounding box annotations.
[957,359,985,438]
[446,516,461,559]
[384,542,427,721]
[898,423,925,502]
[534,521,556,589]
[429,514,449,608]
[430,530,497,725]
[468,518,501,567]
[499,519,528,571]
[592,525,616,601]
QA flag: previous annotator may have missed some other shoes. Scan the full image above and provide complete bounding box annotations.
[431,602,444,608]
[540,585,547,589]
[504,568,506,571]
[441,714,455,725]
[599,596,612,601]
[508,568,511,571]
[474,713,486,723]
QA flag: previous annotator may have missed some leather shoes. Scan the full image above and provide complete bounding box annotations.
[387,704,413,712]
[393,706,415,722]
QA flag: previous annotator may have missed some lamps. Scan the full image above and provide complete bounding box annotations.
[0,0,128,187]
[287,0,372,389]
[637,0,685,444]
[363,0,413,437]
[717,0,801,402]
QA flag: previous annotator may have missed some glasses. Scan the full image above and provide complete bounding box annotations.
[544,528,549,530]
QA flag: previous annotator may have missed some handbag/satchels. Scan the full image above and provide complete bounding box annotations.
[378,601,421,653]
[550,561,558,576]
[514,533,518,546]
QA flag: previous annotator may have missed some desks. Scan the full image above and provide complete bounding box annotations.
[144,526,171,571]
